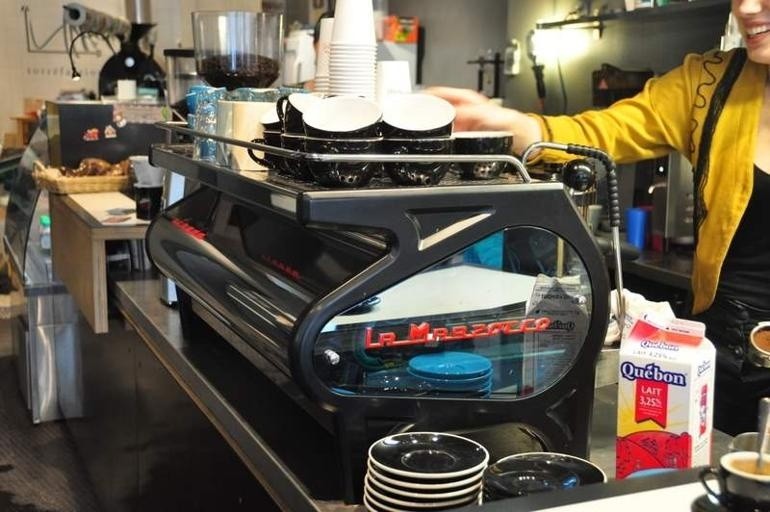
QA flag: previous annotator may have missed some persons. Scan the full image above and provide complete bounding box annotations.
[415,0,770,439]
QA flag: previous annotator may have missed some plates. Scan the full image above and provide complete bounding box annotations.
[406,349,493,399]
[691,490,732,512]
[363,431,490,512]
[482,452,607,504]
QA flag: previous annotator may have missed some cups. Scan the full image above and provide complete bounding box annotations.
[623,205,649,252]
[129,155,167,220]
[697,450,770,512]
[587,204,602,235]
[187,86,513,188]
[313,0,412,102]
